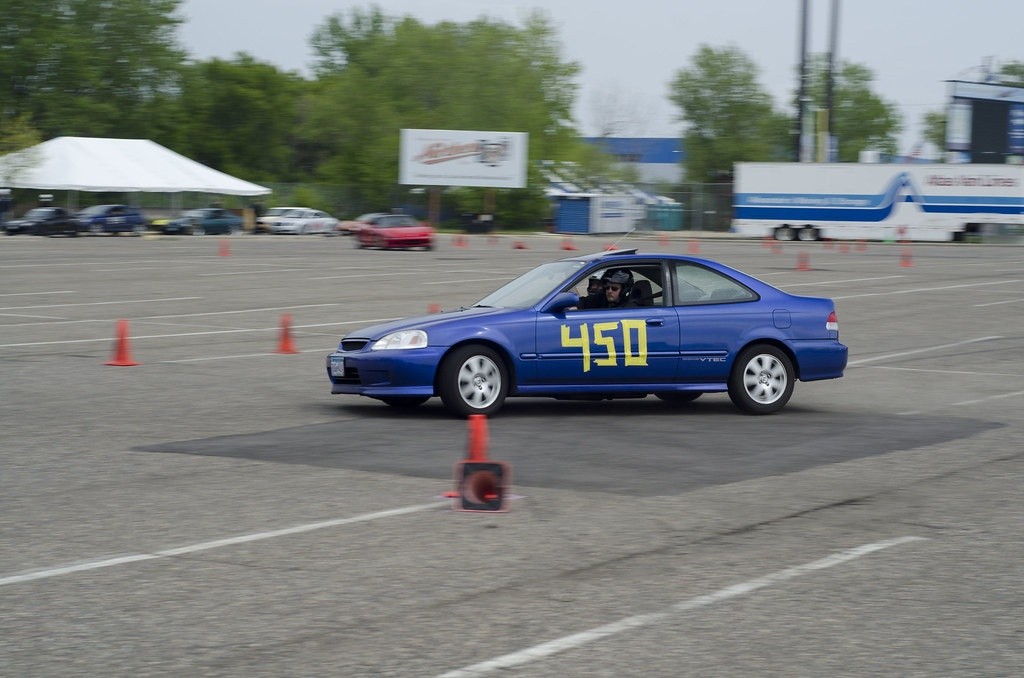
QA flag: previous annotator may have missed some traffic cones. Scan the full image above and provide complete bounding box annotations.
[792,253,812,271]
[446,411,510,512]
[272,315,300,354]
[219,241,230,256]
[897,250,913,268]
[101,317,138,367]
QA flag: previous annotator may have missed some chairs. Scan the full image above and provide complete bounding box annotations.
[630,280,654,307]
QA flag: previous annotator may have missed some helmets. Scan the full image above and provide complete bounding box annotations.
[601,268,635,299]
[586,271,606,296]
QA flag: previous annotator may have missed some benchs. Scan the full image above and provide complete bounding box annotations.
[679,287,751,302]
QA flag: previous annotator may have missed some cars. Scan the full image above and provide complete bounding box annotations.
[324,249,849,421]
[350,210,434,250]
[76,204,148,238]
[150,208,245,236]
[5,208,82,238]
[254,206,338,238]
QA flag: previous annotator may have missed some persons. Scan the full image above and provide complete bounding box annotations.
[586,271,607,296]
[249,198,263,217]
[575,268,648,309]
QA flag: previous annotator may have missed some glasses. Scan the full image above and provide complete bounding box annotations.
[603,284,622,291]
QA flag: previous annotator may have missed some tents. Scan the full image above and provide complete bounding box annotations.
[0,136,273,215]
